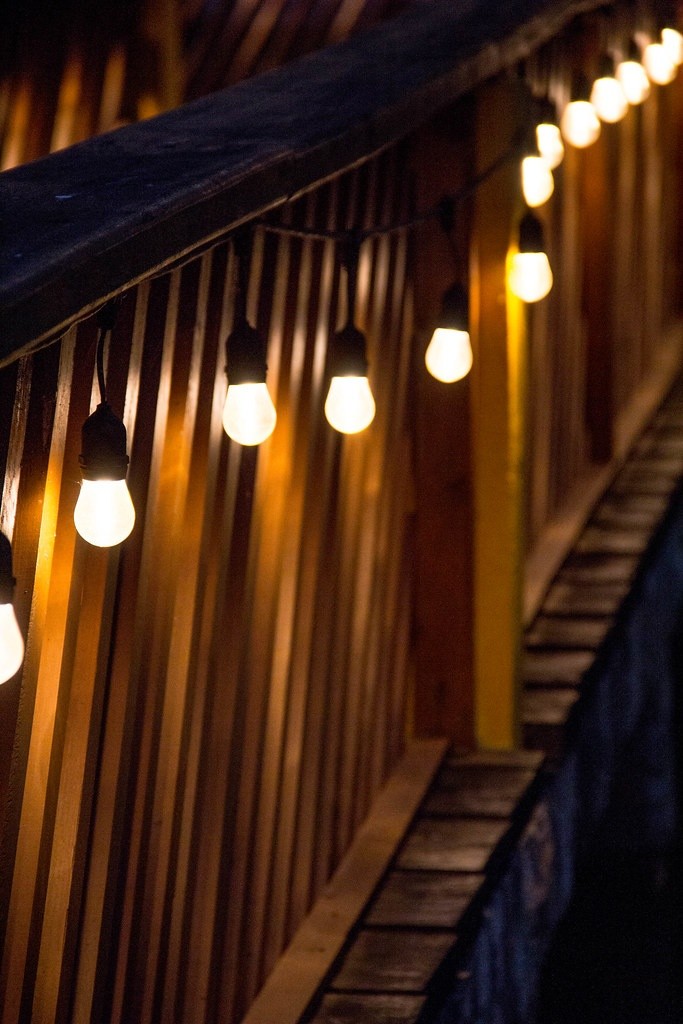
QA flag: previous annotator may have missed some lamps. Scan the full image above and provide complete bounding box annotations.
[423,195,475,386]
[222,223,278,447]
[511,210,552,304]
[0,529,26,687]
[323,231,376,436]
[73,302,135,548]
